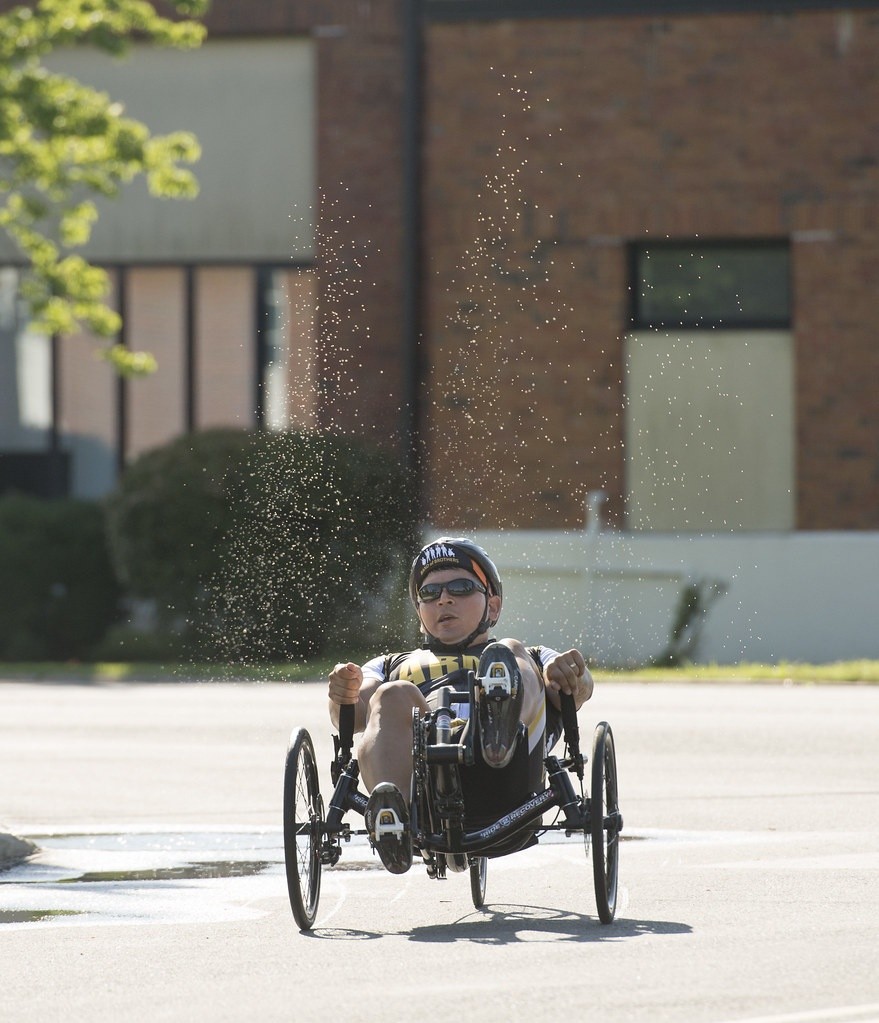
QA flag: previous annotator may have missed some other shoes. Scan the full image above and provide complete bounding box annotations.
[365,782,413,874]
[477,643,524,768]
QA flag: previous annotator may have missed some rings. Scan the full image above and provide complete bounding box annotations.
[570,663,576,668]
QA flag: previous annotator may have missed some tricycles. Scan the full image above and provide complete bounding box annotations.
[279,668,624,932]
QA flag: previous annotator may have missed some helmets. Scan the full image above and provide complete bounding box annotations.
[409,537,503,627]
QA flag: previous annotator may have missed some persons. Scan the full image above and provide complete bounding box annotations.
[328,537,594,874]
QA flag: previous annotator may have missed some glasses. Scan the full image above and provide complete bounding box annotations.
[417,579,488,603]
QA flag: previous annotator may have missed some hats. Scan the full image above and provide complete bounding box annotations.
[416,543,493,597]
